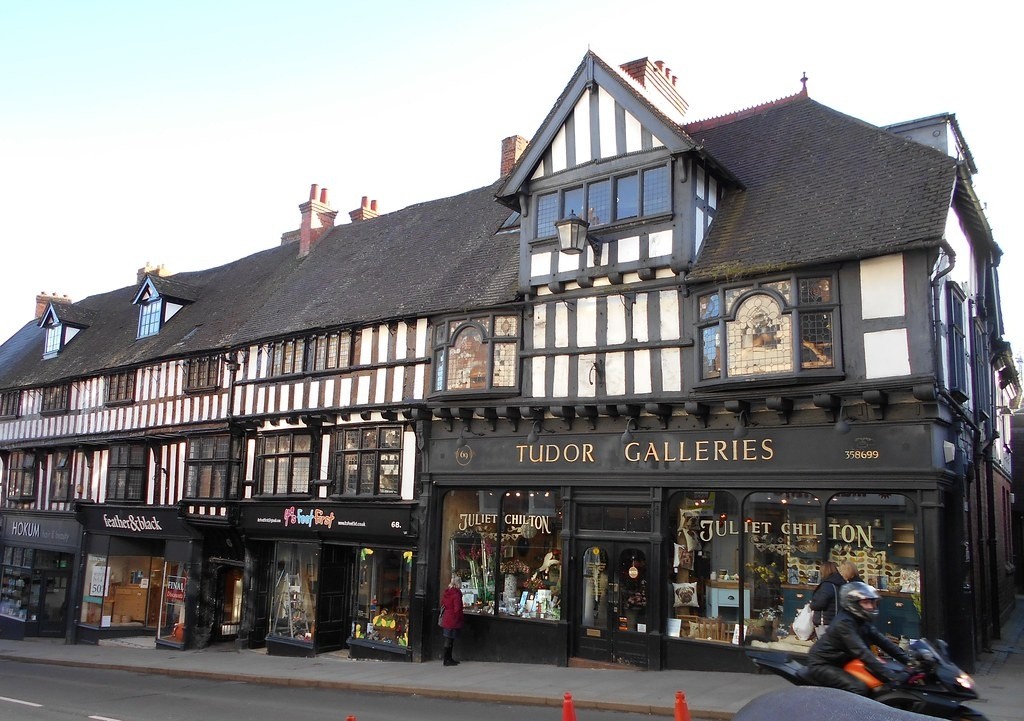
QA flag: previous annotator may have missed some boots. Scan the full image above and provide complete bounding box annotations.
[443,646,461,666]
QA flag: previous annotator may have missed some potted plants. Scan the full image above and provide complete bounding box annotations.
[888,562,903,593]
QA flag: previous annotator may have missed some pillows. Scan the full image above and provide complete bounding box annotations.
[683,529,702,552]
[673,542,695,571]
[672,581,699,608]
[678,509,701,531]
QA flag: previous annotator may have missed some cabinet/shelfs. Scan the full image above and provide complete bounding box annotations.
[705,580,751,619]
[786,521,914,587]
[125,571,144,586]
[449,537,513,575]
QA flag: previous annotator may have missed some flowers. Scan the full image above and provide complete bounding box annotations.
[500,559,529,576]
[524,578,545,592]
[759,608,781,621]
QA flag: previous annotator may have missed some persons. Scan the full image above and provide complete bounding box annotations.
[441,576,464,666]
[807,561,919,699]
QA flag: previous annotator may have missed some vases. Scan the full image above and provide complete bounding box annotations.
[504,574,518,598]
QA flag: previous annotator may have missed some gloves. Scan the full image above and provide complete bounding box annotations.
[888,673,910,684]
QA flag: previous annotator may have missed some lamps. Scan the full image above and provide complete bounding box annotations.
[456,426,485,448]
[733,410,757,438]
[834,406,856,435]
[527,421,556,444]
[554,209,601,267]
[621,418,651,444]
[629,556,639,578]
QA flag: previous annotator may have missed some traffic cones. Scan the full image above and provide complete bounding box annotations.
[673,690,693,721]
[562,692,578,721]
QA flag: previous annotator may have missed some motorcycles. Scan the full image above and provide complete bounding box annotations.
[745,637,992,721]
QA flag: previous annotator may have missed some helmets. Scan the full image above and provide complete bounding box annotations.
[838,581,881,623]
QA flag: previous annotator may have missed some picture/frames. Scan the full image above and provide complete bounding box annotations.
[307,564,314,577]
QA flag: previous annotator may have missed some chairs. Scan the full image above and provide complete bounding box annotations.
[677,615,701,636]
[87,583,116,624]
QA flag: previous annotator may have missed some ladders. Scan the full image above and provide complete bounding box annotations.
[273,573,310,639]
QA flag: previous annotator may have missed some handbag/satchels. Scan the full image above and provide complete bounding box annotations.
[815,625,829,640]
[438,606,444,626]
[792,603,815,641]
